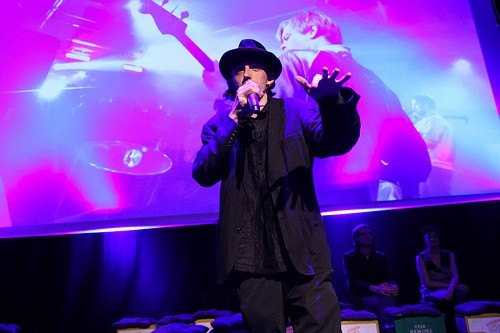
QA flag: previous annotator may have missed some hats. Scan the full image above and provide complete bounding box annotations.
[219,39,282,82]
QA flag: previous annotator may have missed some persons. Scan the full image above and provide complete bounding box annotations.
[415,227,468,308]
[202,10,431,200]
[192,37,361,333]
[408,96,455,197]
[344,223,397,308]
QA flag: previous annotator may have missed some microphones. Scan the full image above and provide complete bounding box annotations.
[248,92,260,113]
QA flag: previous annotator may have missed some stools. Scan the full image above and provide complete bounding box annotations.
[110,300,500,333]
